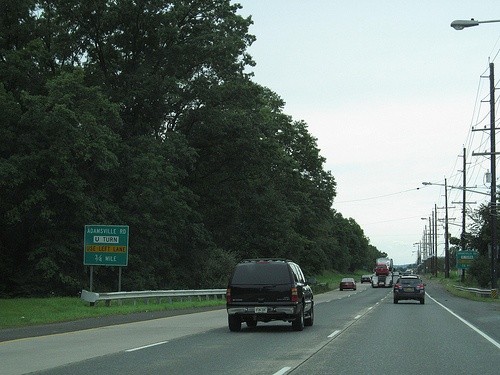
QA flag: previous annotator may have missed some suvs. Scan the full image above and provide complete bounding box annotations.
[225,258,317,333]
[392,274,427,306]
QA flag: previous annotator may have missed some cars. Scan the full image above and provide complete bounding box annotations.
[339,277,357,292]
[360,275,372,284]
[393,269,420,278]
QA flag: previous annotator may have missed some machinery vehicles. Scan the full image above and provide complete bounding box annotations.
[371,257,395,288]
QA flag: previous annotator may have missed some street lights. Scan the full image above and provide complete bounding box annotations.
[421,62,499,289]
[414,211,435,278]
[450,16,500,31]
[421,147,467,282]
